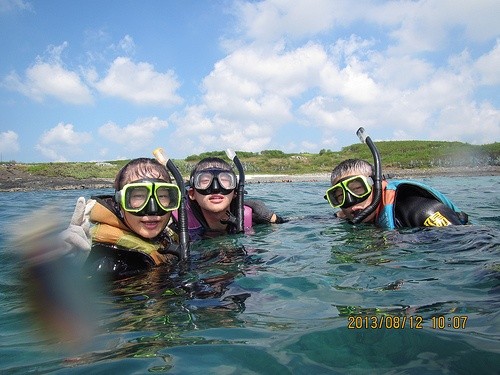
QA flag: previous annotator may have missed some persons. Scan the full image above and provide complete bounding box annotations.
[162,157,291,249]
[55,157,198,288]
[324,158,475,231]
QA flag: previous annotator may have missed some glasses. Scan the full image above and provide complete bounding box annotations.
[325,175,374,209]
[116,178,181,216]
[190,167,238,195]
